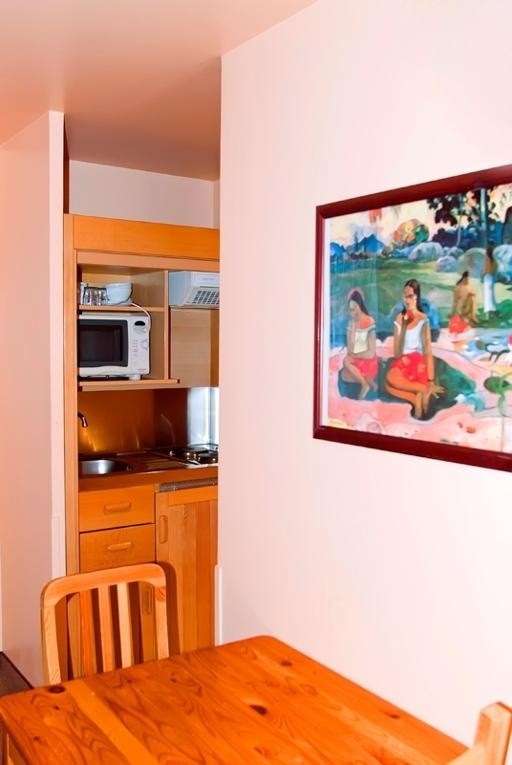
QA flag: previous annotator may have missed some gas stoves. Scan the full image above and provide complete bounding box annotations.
[150,444,218,465]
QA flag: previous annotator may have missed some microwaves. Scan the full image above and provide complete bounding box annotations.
[78,311,151,380]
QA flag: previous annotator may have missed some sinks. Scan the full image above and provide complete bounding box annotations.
[189,452,218,466]
[78,458,134,475]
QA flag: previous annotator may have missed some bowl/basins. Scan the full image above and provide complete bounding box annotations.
[105,282,132,301]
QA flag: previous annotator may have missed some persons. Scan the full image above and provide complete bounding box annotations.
[383,279,448,419]
[480,246,497,312]
[452,271,481,324]
[340,288,381,400]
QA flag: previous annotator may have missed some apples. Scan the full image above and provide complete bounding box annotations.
[403,310,413,321]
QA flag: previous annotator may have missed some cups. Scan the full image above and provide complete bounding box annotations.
[82,287,107,306]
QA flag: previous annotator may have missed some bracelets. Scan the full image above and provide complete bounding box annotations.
[428,379,434,383]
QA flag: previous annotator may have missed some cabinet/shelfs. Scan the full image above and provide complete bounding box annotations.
[79,484,218,669]
[78,271,179,393]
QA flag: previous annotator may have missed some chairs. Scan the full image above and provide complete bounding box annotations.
[448,702,512,765]
[40,563,170,685]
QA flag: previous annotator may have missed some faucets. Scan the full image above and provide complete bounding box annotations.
[78,412,88,428]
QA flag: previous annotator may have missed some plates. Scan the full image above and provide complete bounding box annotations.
[107,301,132,306]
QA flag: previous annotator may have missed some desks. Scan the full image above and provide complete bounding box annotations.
[0,636,469,765]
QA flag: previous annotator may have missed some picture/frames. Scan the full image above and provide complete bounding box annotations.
[312,163,512,473]
